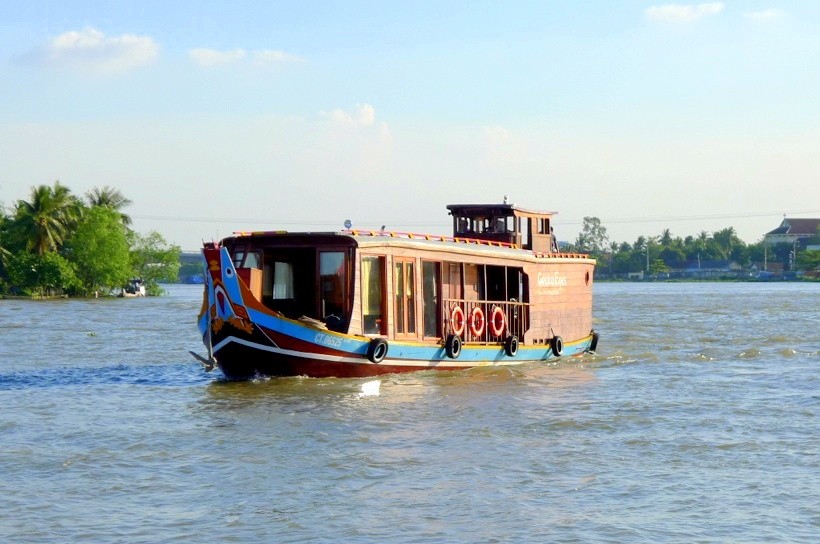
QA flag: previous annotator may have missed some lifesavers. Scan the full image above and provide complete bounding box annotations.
[505,334,518,357]
[489,306,504,338]
[468,307,485,337]
[589,333,600,350]
[450,306,465,334]
[445,334,462,359]
[552,336,563,356]
[367,338,387,364]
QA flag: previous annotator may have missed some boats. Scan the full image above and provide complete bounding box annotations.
[122,276,146,297]
[187,195,600,381]
